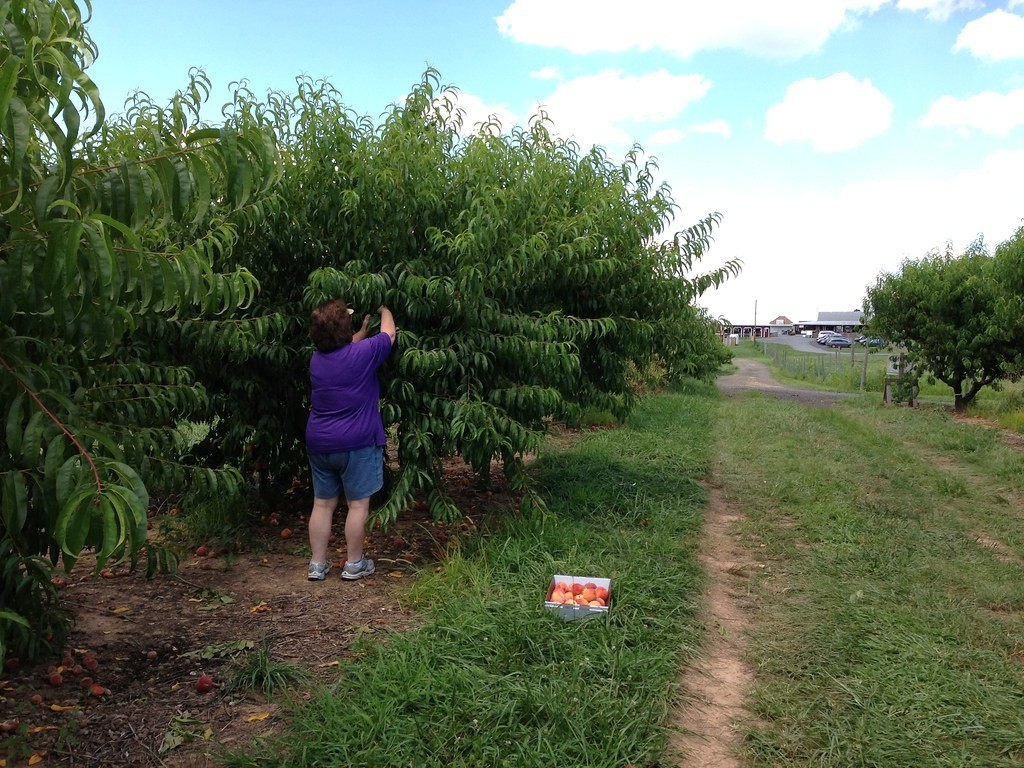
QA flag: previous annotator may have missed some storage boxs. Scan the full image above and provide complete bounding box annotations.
[544,574,612,624]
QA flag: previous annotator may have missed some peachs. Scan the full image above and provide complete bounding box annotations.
[0,475,524,732]
[550,581,609,606]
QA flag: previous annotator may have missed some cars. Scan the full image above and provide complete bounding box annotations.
[817,330,853,348]
[853,333,889,347]
[782,329,797,333]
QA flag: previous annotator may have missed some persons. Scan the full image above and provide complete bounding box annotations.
[306,300,395,581]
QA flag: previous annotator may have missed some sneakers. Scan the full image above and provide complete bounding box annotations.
[307,559,330,581]
[341,553,375,580]
